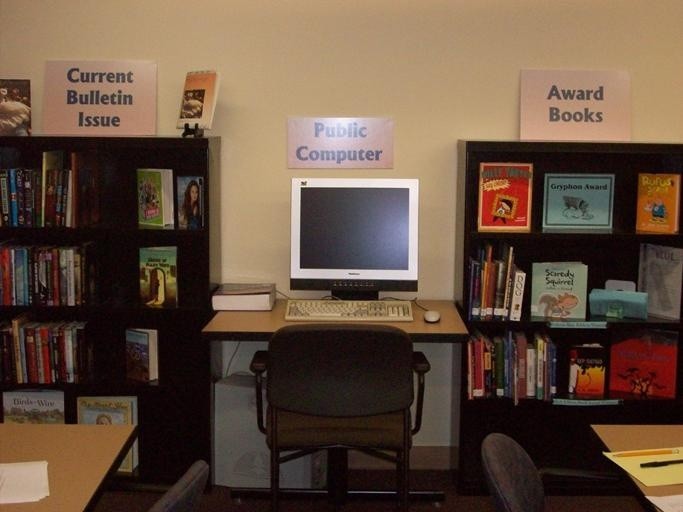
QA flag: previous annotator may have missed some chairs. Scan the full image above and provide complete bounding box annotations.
[250,325,430,512]
[480,433,544,512]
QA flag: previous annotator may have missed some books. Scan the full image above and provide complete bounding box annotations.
[1,390,66,425]
[467,243,681,321]
[636,174,679,234]
[136,167,174,228]
[466,326,676,406]
[478,162,533,233]
[176,176,205,231]
[0,149,98,386]
[211,282,278,312]
[77,396,139,474]
[125,328,159,383]
[175,67,220,131]
[542,174,616,235]
[0,74,33,136]
[139,245,179,308]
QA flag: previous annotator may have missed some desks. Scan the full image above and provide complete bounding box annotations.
[0,424,138,512]
[201,299,470,512]
[590,424,683,512]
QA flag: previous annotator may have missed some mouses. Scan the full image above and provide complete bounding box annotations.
[424,310,442,324]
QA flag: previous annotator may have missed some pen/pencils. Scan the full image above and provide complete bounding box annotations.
[640,459,683,468]
[612,449,680,457]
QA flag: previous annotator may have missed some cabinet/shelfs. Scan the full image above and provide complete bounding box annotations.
[450,140,683,495]
[0,135,222,494]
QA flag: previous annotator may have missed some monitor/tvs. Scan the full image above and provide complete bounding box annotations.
[289,177,419,301]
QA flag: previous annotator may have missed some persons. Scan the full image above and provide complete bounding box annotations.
[181,179,200,230]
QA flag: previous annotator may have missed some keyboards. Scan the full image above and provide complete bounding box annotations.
[284,301,414,323]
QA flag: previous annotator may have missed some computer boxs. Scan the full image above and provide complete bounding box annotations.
[215,374,328,490]
